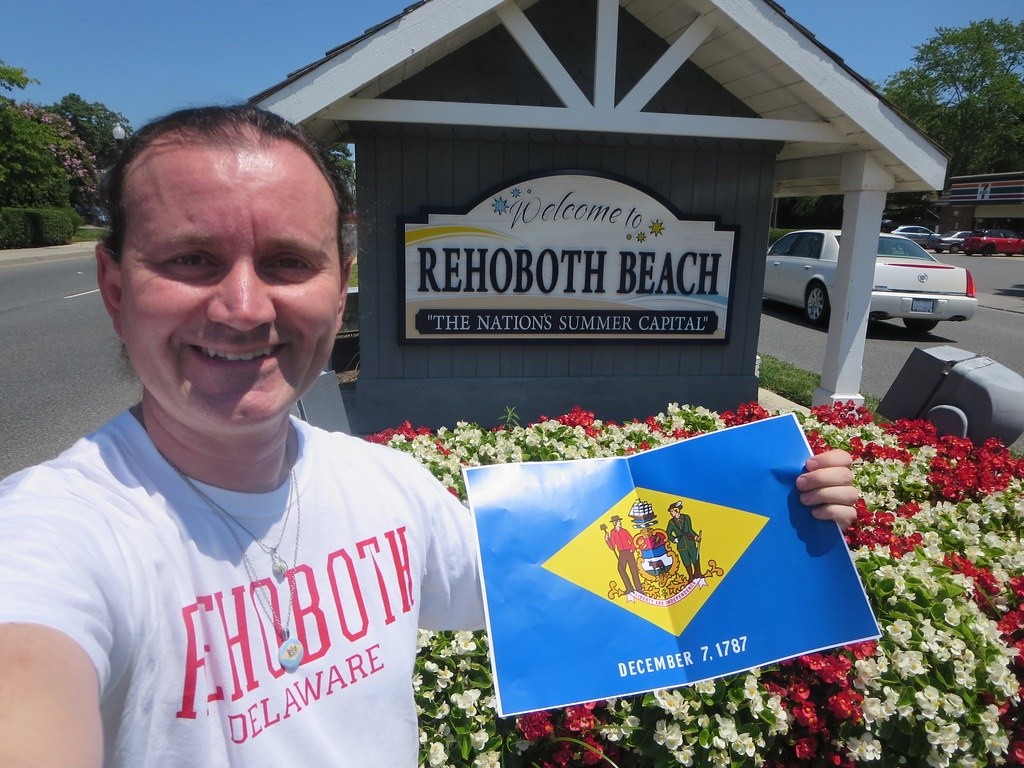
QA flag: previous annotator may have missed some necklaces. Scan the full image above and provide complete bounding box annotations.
[161,442,303,668]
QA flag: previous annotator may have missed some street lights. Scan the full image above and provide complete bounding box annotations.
[111,121,125,159]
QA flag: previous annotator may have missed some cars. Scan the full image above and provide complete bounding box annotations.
[762,229,978,332]
[929,231,973,254]
[964,229,1024,257]
[890,225,942,249]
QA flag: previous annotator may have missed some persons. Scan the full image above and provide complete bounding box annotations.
[0,104,860,768]
